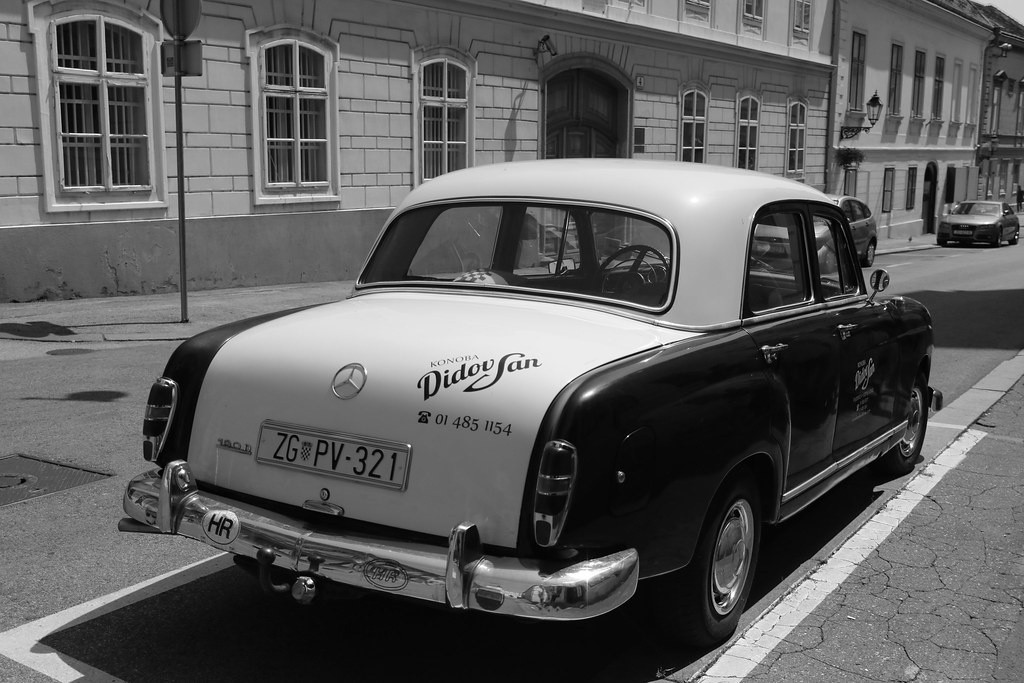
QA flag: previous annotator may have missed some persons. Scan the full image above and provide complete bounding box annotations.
[1017,185,1023,212]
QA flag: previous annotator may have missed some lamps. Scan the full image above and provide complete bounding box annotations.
[839,90,884,141]
[978,131,999,162]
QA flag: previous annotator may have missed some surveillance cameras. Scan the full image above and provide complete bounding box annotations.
[541,34,558,57]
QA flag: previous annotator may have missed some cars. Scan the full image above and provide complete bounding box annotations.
[937,198,1020,248]
[118,159,943,647]
[751,195,877,273]
